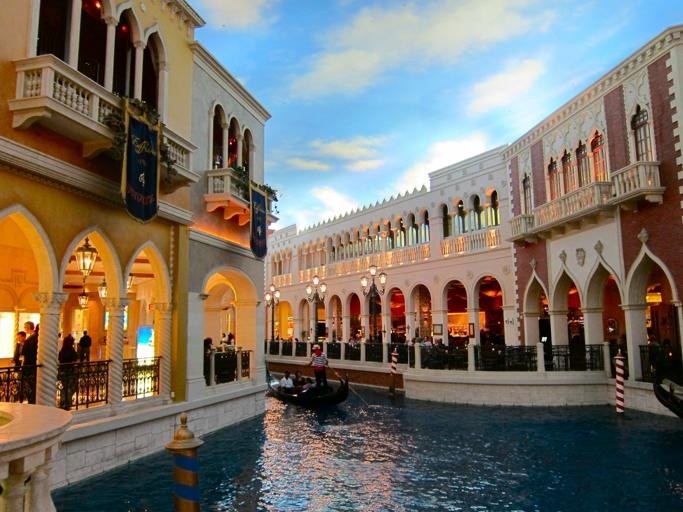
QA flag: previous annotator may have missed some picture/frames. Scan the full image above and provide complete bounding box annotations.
[468,322,474,338]
[431,323,443,336]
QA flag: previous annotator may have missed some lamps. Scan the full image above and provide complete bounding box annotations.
[74,235,98,280]
[76,277,89,308]
[126,271,134,289]
[96,273,107,305]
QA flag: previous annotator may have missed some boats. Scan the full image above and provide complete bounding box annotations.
[264,359,348,407]
[653,351,683,420]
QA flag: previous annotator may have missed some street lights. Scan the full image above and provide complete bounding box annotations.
[264,282,280,355]
[305,274,327,344]
[359,264,386,361]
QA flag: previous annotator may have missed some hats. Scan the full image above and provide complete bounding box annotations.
[312,345,320,350]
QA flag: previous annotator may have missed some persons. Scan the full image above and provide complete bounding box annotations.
[480,329,486,345]
[279,343,328,394]
[204,337,213,353]
[13,320,92,410]
[335,331,448,350]
[647,335,663,377]
[220,332,235,344]
